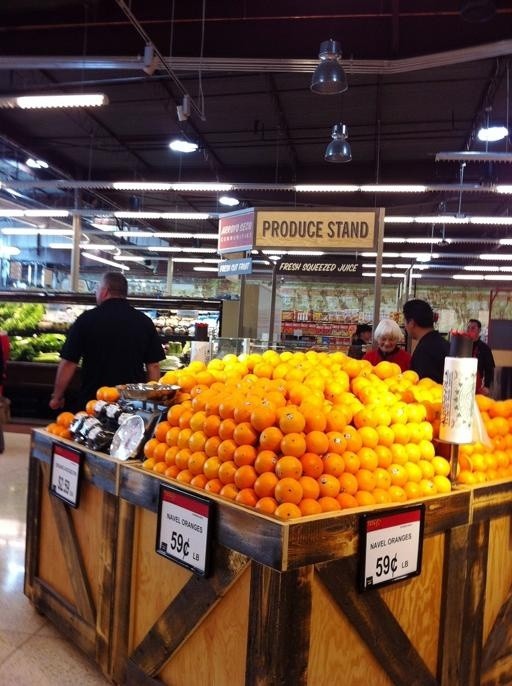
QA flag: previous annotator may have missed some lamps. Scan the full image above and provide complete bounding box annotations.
[310,36,348,94]
[182,94,190,117]
[177,105,188,122]
[143,42,154,67]
[324,122,352,163]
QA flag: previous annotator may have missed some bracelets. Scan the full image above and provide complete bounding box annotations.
[51,394,65,402]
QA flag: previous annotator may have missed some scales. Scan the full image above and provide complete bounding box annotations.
[109,382,182,462]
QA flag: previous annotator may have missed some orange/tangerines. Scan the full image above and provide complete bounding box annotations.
[45,350,512,522]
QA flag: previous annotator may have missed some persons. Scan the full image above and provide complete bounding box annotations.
[466,318,496,394]
[348,322,372,360]
[403,299,449,386]
[49,271,167,415]
[0,330,10,453]
[362,319,414,374]
